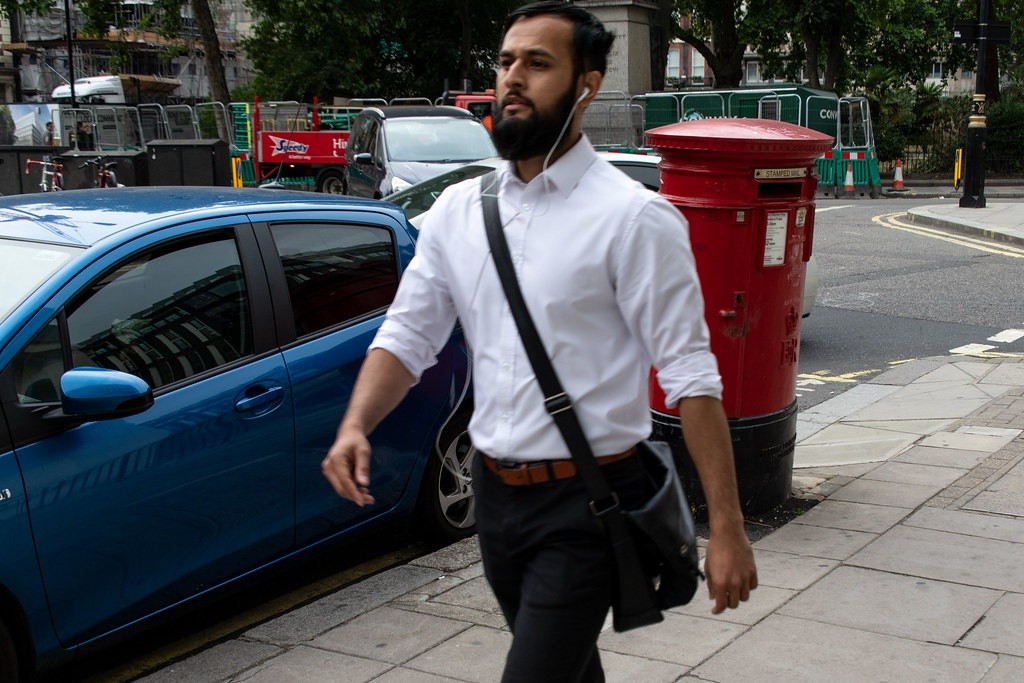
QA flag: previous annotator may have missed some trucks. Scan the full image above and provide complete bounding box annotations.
[251,96,496,196]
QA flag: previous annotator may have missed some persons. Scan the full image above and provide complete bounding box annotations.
[324,0,759,683]
[43,122,96,150]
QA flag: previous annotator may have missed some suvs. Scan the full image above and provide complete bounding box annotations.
[345,104,499,198]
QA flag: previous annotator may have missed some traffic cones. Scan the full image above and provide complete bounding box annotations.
[839,164,859,200]
[886,158,911,191]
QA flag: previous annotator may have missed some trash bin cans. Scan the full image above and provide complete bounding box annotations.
[145,138,232,187]
[60,150,148,190]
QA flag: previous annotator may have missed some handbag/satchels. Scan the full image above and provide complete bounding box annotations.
[592,440,708,634]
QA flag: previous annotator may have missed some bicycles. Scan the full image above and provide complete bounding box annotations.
[25,159,64,192]
[78,155,126,187]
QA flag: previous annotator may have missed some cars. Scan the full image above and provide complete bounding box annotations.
[1,187,479,683]
[596,151,820,317]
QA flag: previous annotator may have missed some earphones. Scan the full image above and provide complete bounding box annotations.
[577,86,591,102]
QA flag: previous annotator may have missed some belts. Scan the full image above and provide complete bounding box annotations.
[484,445,635,486]
